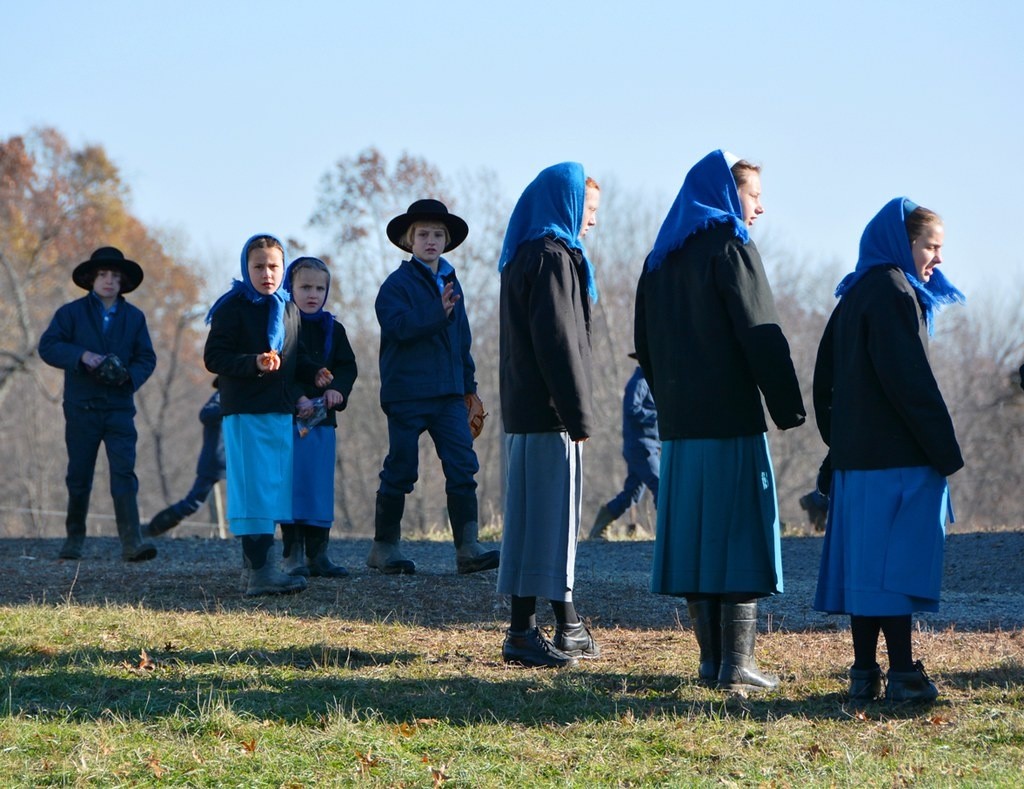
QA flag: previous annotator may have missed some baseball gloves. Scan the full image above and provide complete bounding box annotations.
[90,354,129,388]
[465,394,484,439]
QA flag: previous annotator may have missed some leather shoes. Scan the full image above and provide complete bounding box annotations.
[884,660,937,703]
[502,626,574,666]
[848,663,882,698]
[555,615,600,661]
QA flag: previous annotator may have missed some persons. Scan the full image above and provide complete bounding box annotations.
[366,199,500,573]
[495,161,601,668]
[634,148,806,692]
[139,376,226,537]
[282,257,358,578]
[589,353,661,544]
[38,246,158,561]
[204,234,334,595]
[798,449,832,532]
[812,197,966,705]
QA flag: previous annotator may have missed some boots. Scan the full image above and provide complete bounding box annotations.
[280,524,309,578]
[237,555,250,593]
[687,599,723,681]
[116,498,157,562]
[60,496,88,559]
[719,602,779,693]
[448,492,500,574]
[588,507,613,542]
[367,492,416,575]
[305,527,346,576]
[142,506,184,538]
[243,536,308,596]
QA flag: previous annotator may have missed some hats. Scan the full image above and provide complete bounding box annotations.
[387,200,468,253]
[73,247,143,294]
